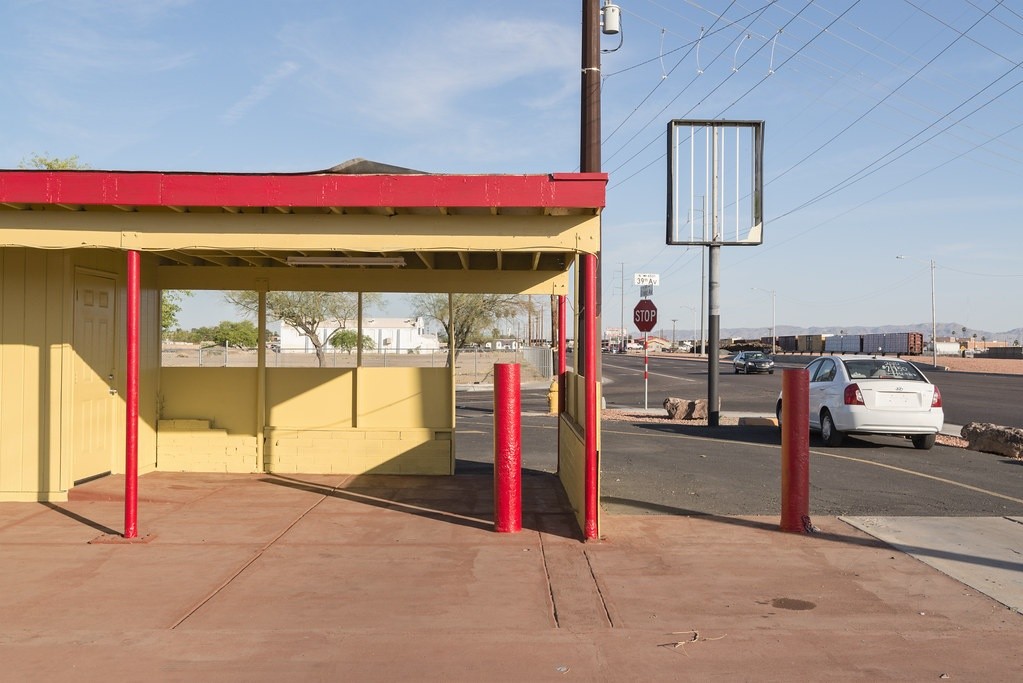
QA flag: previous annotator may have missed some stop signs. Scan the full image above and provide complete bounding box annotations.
[633,300,657,332]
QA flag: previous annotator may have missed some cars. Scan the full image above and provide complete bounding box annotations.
[732,351,775,375]
[776,354,945,450]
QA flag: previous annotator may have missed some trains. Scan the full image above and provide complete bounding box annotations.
[678,332,923,358]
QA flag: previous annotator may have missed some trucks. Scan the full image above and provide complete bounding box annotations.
[959,349,974,358]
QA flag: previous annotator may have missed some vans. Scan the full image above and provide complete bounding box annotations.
[627,343,643,350]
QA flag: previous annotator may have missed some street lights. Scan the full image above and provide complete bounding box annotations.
[896,255,936,368]
[750,288,776,354]
